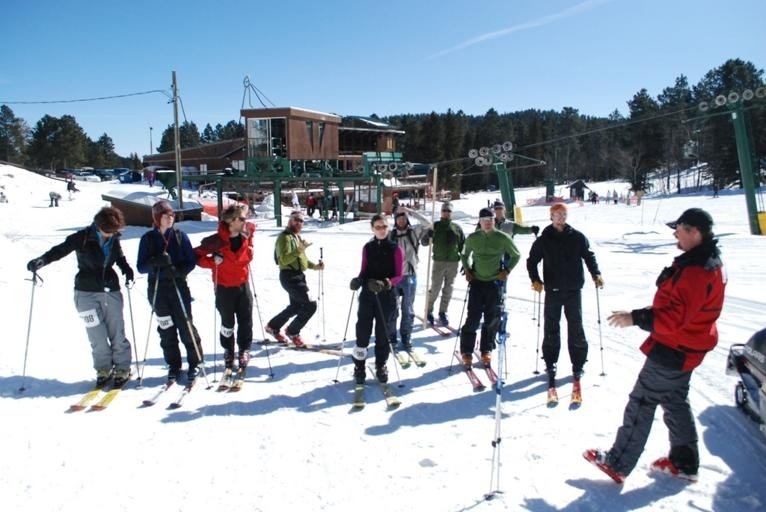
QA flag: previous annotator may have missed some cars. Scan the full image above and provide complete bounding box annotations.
[51,165,144,184]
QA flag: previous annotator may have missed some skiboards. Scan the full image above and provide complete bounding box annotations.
[254,338,352,357]
[353,363,402,407]
[454,348,504,389]
[389,341,426,368]
[143,379,193,409]
[548,377,582,407]
[70,368,134,408]
[414,311,461,336]
[219,363,247,392]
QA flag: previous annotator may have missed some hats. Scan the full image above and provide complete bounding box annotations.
[153,201,173,228]
[666,209,712,229]
[290,211,305,222]
[394,205,412,227]
[442,200,453,212]
[551,204,567,221]
[479,202,504,219]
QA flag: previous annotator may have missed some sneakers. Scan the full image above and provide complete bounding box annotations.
[427,311,449,324]
[583,449,626,483]
[546,360,557,375]
[168,366,181,379]
[481,351,491,363]
[224,352,233,367]
[354,366,366,377]
[114,369,130,384]
[573,362,583,378]
[265,325,285,341]
[285,328,304,345]
[238,351,250,367]
[189,363,200,377]
[651,458,698,482]
[462,353,473,365]
[389,333,412,347]
[97,369,113,382]
[375,363,388,377]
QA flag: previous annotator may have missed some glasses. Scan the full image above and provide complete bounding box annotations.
[233,217,247,223]
[375,225,388,230]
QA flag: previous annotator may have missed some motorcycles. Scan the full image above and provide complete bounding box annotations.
[725,326,765,427]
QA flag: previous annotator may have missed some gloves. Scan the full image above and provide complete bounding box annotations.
[397,206,413,213]
[152,253,171,264]
[532,281,543,292]
[592,274,604,286]
[314,261,324,270]
[465,268,474,282]
[350,277,363,290]
[498,268,509,282]
[214,251,223,265]
[27,256,47,272]
[124,267,133,279]
[298,238,312,253]
[427,229,434,237]
[368,278,391,292]
[531,226,539,233]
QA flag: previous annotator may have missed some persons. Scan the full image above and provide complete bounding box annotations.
[636,187,644,207]
[393,192,401,211]
[383,207,432,346]
[136,201,198,379]
[347,214,404,382]
[588,188,618,204]
[459,209,520,371]
[421,202,465,326]
[68,180,79,200]
[476,200,539,347]
[713,182,722,199]
[192,204,257,368]
[583,208,728,485]
[27,207,134,386]
[148,169,155,186]
[263,209,324,346]
[49,191,61,207]
[285,187,338,223]
[528,203,604,378]
